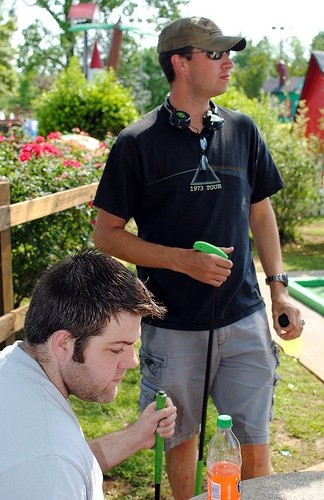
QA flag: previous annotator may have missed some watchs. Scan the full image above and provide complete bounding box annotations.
[265,273,288,287]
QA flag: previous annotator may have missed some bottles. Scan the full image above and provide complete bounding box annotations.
[206,414,242,500]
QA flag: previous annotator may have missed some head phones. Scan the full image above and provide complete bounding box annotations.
[164,91,225,131]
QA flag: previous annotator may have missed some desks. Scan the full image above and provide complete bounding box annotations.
[188,470,324,500]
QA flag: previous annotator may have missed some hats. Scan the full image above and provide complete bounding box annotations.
[157,16,247,52]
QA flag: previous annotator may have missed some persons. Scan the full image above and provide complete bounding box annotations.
[93,16,307,500]
[0,246,168,500]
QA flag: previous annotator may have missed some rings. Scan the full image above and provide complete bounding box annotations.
[302,320,305,325]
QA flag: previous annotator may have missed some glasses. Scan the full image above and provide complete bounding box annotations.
[181,49,232,60]
